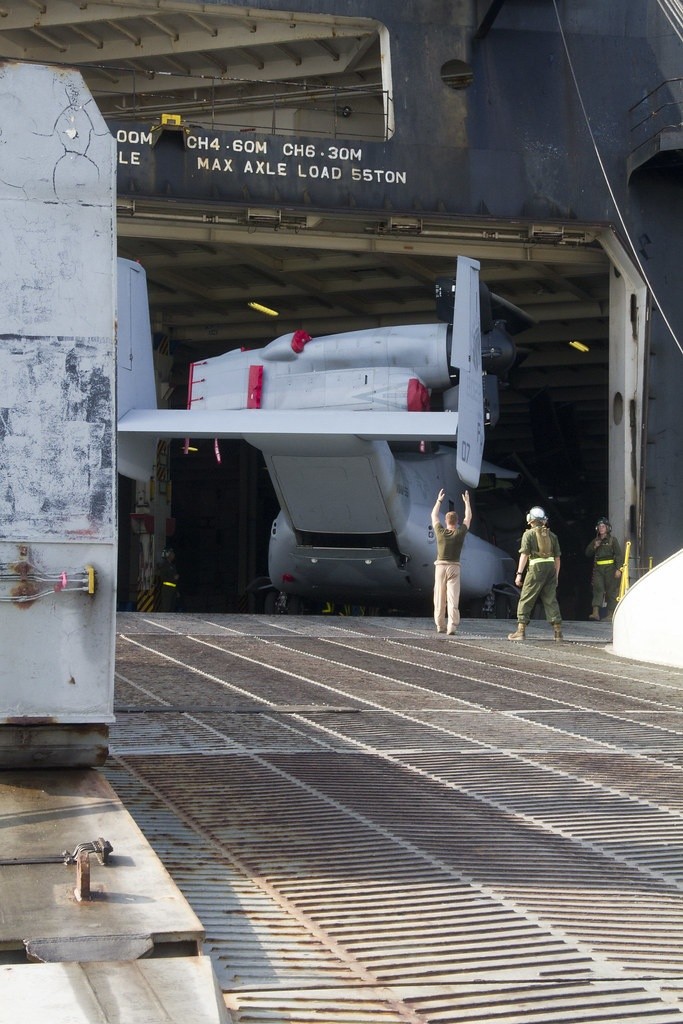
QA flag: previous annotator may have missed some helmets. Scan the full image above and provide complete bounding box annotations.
[595,517,612,532]
[527,506,548,524]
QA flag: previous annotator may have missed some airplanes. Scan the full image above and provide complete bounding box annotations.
[116,255,518,619]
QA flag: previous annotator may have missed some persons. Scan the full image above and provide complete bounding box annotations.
[508,507,563,642]
[585,517,624,621]
[431,488,473,634]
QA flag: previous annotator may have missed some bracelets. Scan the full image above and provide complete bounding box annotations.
[516,571,523,575]
[437,499,442,504]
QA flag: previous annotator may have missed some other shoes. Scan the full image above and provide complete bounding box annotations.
[590,613,601,620]
[554,630,563,642]
[447,629,456,635]
[437,626,446,633]
[508,633,525,640]
